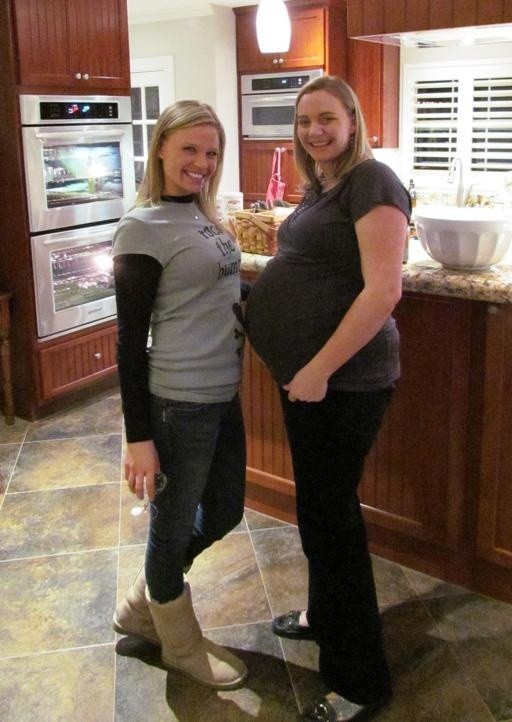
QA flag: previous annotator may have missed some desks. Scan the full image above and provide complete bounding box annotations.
[0,290,16,426]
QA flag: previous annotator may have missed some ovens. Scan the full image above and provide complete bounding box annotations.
[239,69,324,142]
[18,93,138,343]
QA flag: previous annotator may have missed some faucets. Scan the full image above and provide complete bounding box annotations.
[447,156,465,208]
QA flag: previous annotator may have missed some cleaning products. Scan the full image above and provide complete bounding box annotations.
[407,178,417,208]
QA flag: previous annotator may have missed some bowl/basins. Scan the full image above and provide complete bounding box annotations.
[412,205,511,272]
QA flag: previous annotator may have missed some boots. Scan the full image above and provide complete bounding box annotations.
[112,562,194,647]
[144,572,249,690]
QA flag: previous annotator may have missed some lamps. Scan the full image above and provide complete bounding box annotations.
[254,0,292,55]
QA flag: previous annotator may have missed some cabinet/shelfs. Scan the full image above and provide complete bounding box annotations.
[237,0,347,83]
[7,325,120,422]
[239,140,313,206]
[346,2,401,149]
[347,1,512,48]
[1,0,131,91]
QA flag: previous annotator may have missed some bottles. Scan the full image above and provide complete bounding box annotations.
[407,179,417,210]
[266,147,286,207]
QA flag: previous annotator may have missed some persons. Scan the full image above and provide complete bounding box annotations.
[244,76,412,722]
[111,101,252,691]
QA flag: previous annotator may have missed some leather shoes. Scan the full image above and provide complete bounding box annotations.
[298,690,393,721]
[272,609,315,641]
